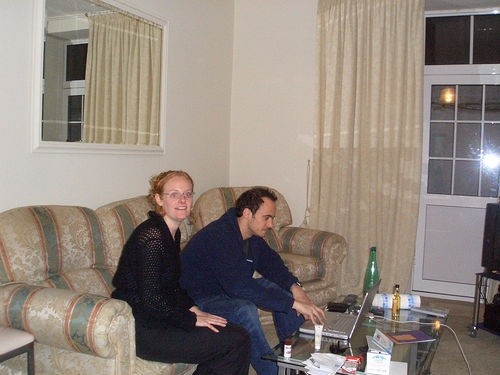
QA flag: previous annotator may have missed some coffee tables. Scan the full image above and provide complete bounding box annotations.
[260,294,451,375]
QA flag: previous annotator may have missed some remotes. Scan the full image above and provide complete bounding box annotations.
[410,306,447,318]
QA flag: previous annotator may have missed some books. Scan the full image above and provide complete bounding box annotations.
[382,329,436,345]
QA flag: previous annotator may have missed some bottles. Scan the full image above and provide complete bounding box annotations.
[363,246,380,313]
[392,284,401,321]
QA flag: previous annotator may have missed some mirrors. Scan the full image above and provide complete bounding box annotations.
[31,0,169,153]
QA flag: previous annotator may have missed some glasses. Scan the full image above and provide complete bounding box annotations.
[160,192,195,199]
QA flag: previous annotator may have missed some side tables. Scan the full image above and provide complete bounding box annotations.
[0,325,35,375]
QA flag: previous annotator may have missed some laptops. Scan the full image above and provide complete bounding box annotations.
[299,279,382,339]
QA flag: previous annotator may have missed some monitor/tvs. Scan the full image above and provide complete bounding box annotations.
[482,203,500,273]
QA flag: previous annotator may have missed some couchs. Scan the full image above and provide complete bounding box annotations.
[0,187,347,375]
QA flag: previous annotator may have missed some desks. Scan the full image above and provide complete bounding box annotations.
[468,272,500,337]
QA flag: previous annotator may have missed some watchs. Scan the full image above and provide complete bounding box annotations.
[295,281,303,287]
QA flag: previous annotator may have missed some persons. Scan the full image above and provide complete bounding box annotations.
[110,170,252,375]
[179,186,327,375]
